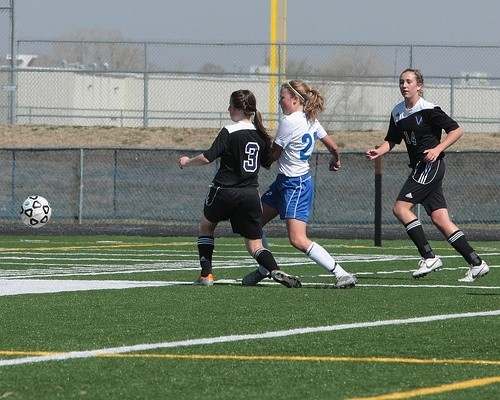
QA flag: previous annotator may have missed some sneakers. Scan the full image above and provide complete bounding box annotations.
[412,254,443,279]
[335,272,358,289]
[242,268,270,286]
[271,270,302,288]
[457,259,490,282]
[192,274,213,286]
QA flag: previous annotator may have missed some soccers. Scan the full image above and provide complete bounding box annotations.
[19,194,52,228]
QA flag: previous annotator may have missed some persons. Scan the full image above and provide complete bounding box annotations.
[366,69,490,282]
[179,90,302,288]
[242,80,357,288]
